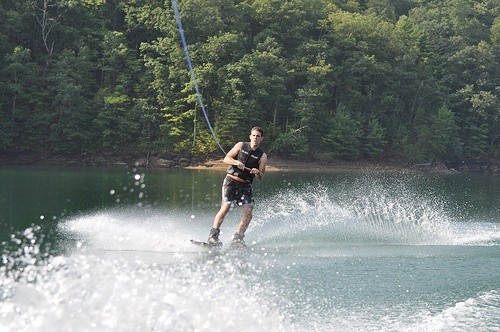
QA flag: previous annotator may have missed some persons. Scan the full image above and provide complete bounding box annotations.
[208,127,268,248]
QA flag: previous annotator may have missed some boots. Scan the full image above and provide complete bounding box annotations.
[232,232,247,250]
[206,228,223,247]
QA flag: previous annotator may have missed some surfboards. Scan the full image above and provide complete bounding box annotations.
[190,238,255,248]
